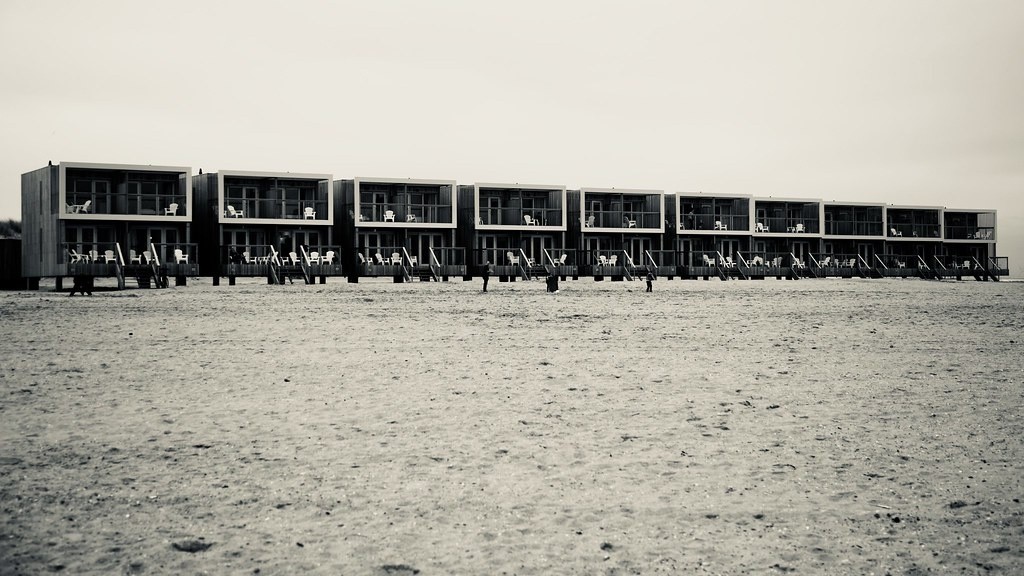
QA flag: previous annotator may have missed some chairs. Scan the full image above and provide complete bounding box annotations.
[891,228,992,270]
[594,255,617,266]
[507,252,519,265]
[407,214,417,223]
[105,250,116,264]
[758,223,768,232]
[375,253,390,266]
[67,250,99,264]
[585,215,595,228]
[227,205,244,218]
[554,254,568,266]
[175,249,189,264]
[383,210,395,222]
[358,253,374,265]
[702,251,856,268]
[524,215,539,226]
[243,249,335,266]
[796,223,805,233]
[66,200,91,214]
[304,207,316,219]
[390,252,403,266]
[715,221,728,230]
[165,203,179,216]
[143,251,155,265]
[410,256,417,266]
[625,217,637,228]
[130,249,142,265]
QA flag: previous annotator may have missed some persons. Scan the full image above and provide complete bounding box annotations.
[482,261,492,293]
[688,209,694,229]
[934,224,940,238]
[646,271,655,292]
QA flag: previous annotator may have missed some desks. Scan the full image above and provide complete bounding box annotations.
[73,205,84,214]
